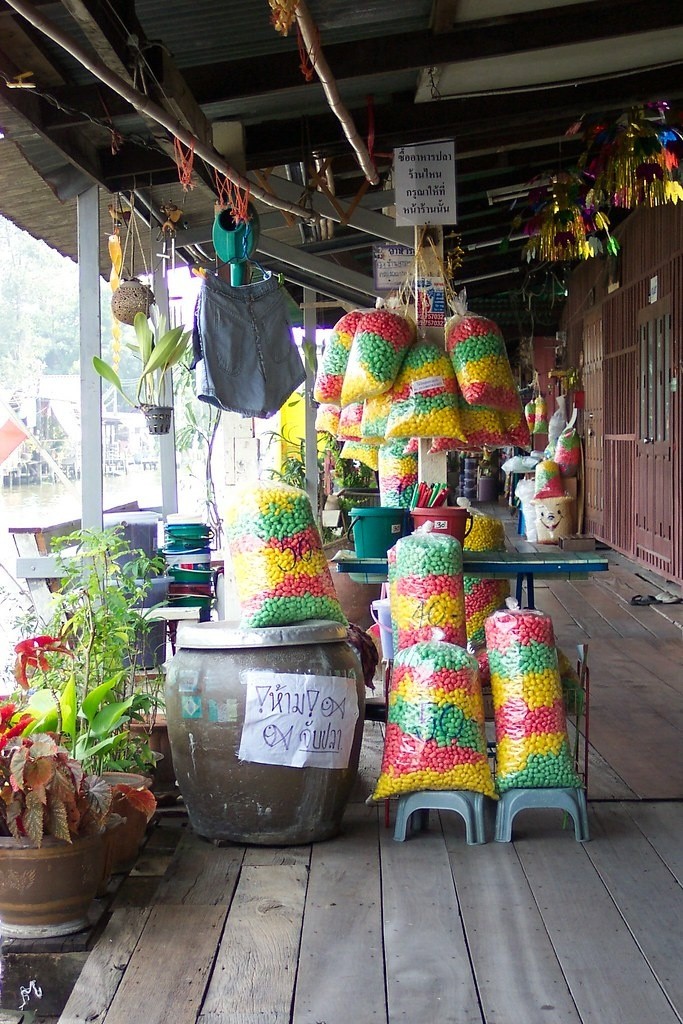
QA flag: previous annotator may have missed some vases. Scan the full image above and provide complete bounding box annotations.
[0,820,109,942]
[126,751,164,795]
[98,772,149,863]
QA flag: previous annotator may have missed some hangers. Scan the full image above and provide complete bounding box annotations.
[213,220,270,281]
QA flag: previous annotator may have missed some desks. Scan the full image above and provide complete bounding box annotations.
[331,548,612,718]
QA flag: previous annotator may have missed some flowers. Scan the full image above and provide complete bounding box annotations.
[0,524,175,848]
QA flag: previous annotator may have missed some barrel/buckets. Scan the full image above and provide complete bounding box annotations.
[410,506,473,553]
[463,458,497,502]
[347,507,409,558]
[103,509,216,670]
[370,597,394,660]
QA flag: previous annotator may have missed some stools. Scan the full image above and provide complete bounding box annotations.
[392,790,486,846]
[494,786,590,843]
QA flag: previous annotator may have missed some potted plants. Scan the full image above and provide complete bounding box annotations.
[91,304,196,436]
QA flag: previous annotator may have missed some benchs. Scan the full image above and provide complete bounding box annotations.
[8,501,224,713]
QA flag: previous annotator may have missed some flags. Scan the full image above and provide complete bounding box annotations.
[0,401,29,466]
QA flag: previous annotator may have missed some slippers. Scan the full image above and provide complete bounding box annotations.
[631,594,663,606]
[655,590,683,603]
